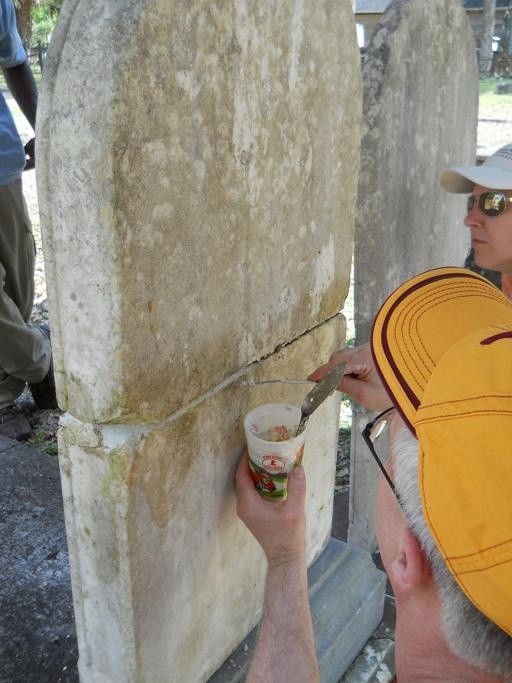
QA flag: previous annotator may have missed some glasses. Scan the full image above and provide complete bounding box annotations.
[466,191,511,217]
[360,407,412,520]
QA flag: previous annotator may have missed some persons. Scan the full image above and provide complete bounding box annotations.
[0,0,57,444]
[438,142,512,296]
[231,263,512,681]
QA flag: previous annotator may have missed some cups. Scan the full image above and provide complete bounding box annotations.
[241,403,307,503]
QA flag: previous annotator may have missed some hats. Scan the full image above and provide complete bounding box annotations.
[370,266,512,636]
[439,144,512,193]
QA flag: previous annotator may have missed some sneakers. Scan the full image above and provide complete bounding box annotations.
[27,326,58,410]
[1,407,32,443]
